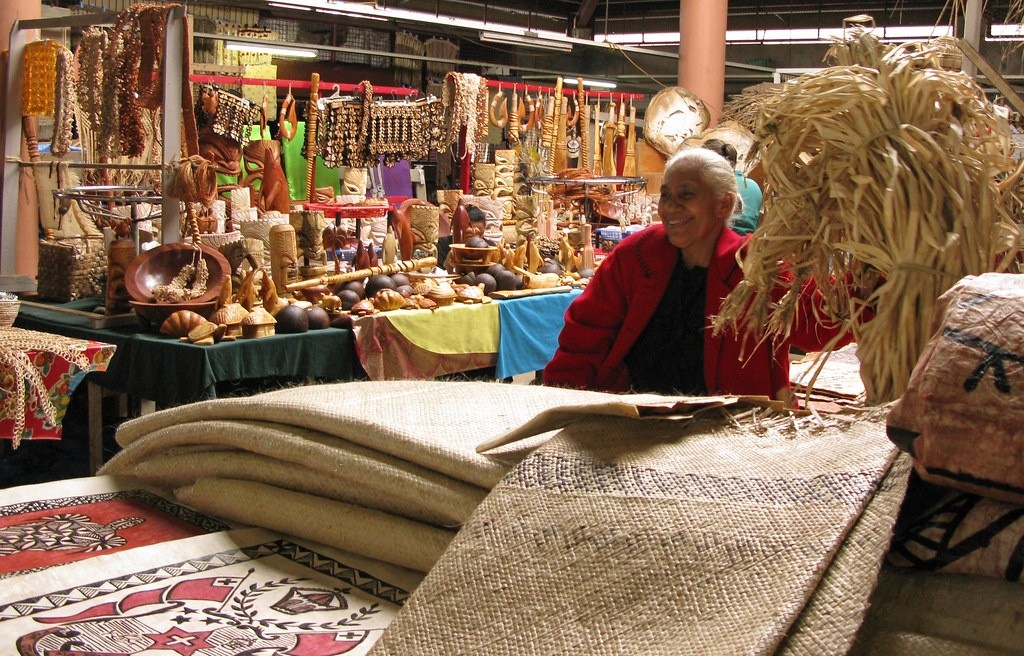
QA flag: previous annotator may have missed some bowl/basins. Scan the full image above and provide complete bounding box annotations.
[125,243,232,329]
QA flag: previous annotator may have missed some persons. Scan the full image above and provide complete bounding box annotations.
[434,206,499,268]
[543,139,878,407]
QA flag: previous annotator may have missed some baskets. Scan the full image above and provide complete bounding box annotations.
[0,300,22,331]
[37,237,109,302]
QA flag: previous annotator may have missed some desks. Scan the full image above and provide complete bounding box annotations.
[0,327,117,478]
[15,294,354,422]
[347,286,585,383]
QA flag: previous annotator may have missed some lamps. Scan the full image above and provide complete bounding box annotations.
[591,0,1024,49]
[479,31,573,53]
[224,41,318,58]
